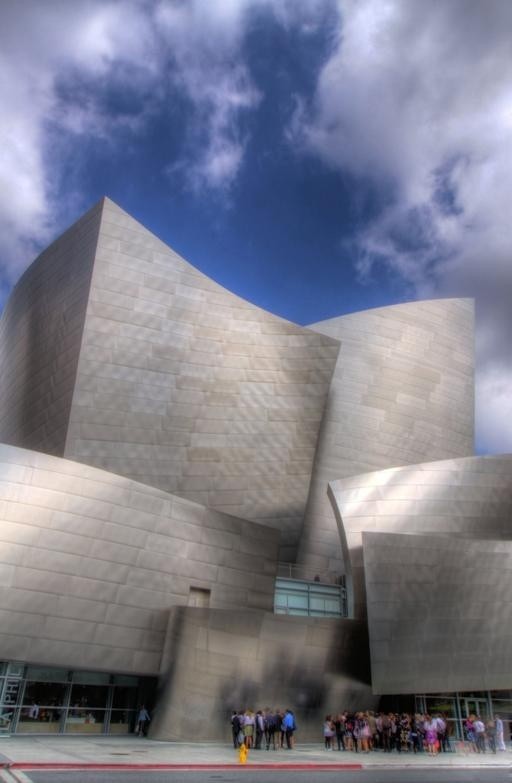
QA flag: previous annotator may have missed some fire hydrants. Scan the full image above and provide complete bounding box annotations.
[239,743,250,763]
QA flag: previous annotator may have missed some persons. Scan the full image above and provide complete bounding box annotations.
[230,704,507,758]
[3,693,90,721]
[136,702,151,737]
